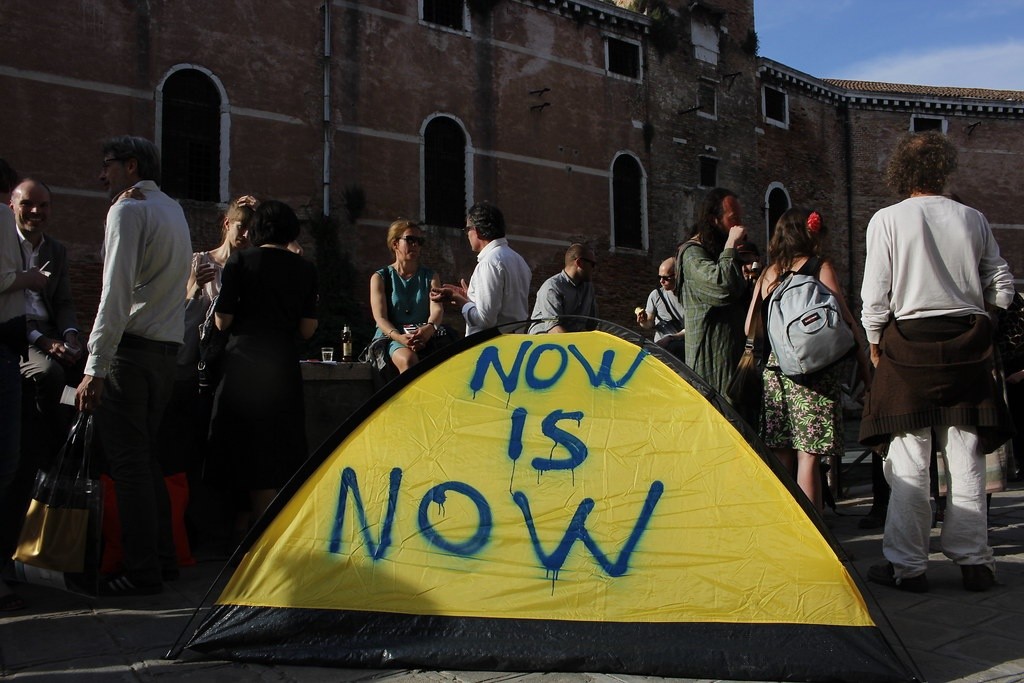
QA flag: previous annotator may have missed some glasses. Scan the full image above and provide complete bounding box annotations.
[462,225,475,235]
[103,158,123,173]
[657,274,674,280]
[575,258,596,268]
[398,235,425,245]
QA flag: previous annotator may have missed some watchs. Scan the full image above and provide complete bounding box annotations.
[428,323,438,331]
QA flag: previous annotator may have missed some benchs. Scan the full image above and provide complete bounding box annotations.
[298,360,375,467]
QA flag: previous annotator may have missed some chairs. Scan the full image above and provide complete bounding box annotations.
[829,355,872,500]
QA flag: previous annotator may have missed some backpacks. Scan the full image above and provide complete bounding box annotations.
[759,255,860,377]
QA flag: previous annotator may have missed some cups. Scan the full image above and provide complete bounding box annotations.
[321,347,334,361]
[64,342,81,355]
[403,328,416,334]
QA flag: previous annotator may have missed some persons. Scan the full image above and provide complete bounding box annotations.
[526,241,598,335]
[743,207,864,511]
[676,187,766,423]
[637,257,684,362]
[368,217,446,378]
[859,130,1024,591]
[429,203,532,335]
[0,136,318,593]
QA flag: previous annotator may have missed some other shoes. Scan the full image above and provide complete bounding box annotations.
[1006,465,1024,485]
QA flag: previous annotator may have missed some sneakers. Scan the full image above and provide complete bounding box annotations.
[960,563,992,591]
[867,561,925,593]
[88,563,163,595]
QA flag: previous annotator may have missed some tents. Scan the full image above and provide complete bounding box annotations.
[163,316,927,683]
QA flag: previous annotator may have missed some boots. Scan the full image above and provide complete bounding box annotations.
[858,495,889,526]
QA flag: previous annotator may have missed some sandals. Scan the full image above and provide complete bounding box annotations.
[0,592,28,611]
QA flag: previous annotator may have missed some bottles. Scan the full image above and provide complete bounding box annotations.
[342,324,352,362]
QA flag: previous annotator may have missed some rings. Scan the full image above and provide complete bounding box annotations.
[197,272,201,275]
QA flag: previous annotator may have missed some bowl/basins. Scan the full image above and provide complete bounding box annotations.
[23,270,52,277]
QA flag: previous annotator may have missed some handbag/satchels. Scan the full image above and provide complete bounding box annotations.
[726,349,768,404]
[14,409,103,600]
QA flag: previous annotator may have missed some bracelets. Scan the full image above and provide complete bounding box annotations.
[388,329,399,341]
